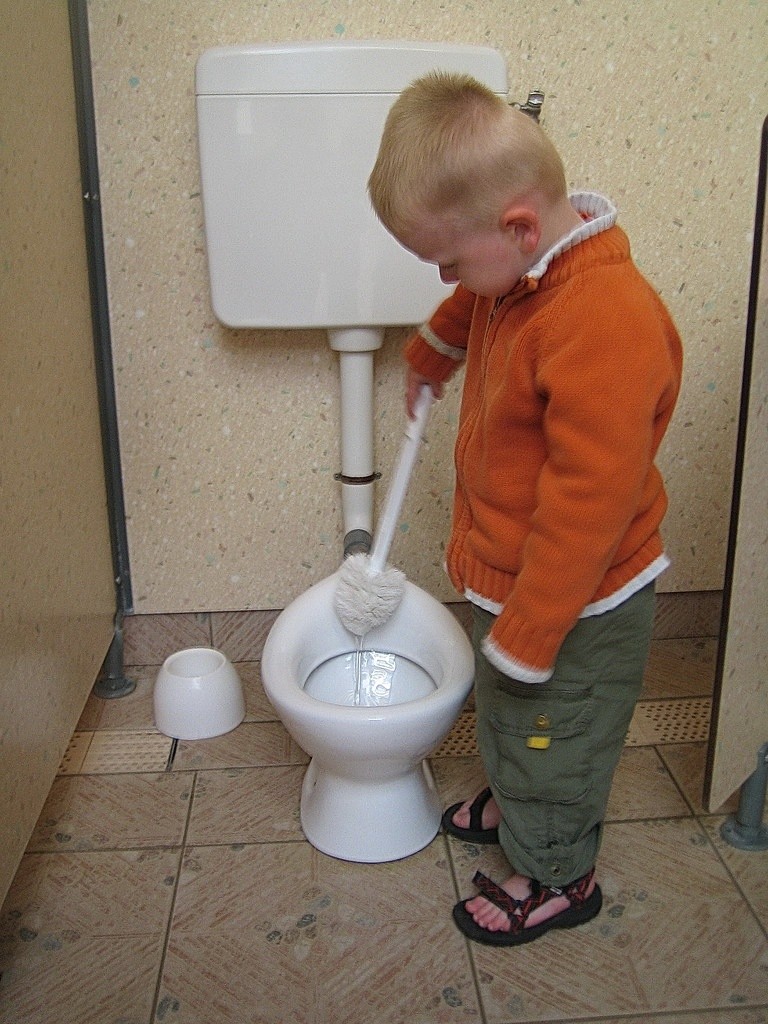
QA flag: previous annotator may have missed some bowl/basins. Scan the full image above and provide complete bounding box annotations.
[154,646,246,741]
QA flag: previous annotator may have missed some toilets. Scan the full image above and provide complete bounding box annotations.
[196,43,511,866]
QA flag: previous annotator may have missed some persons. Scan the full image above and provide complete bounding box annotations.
[365,73,684,950]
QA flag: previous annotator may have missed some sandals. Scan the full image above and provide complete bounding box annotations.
[452,865,604,946]
[441,786,501,844]
